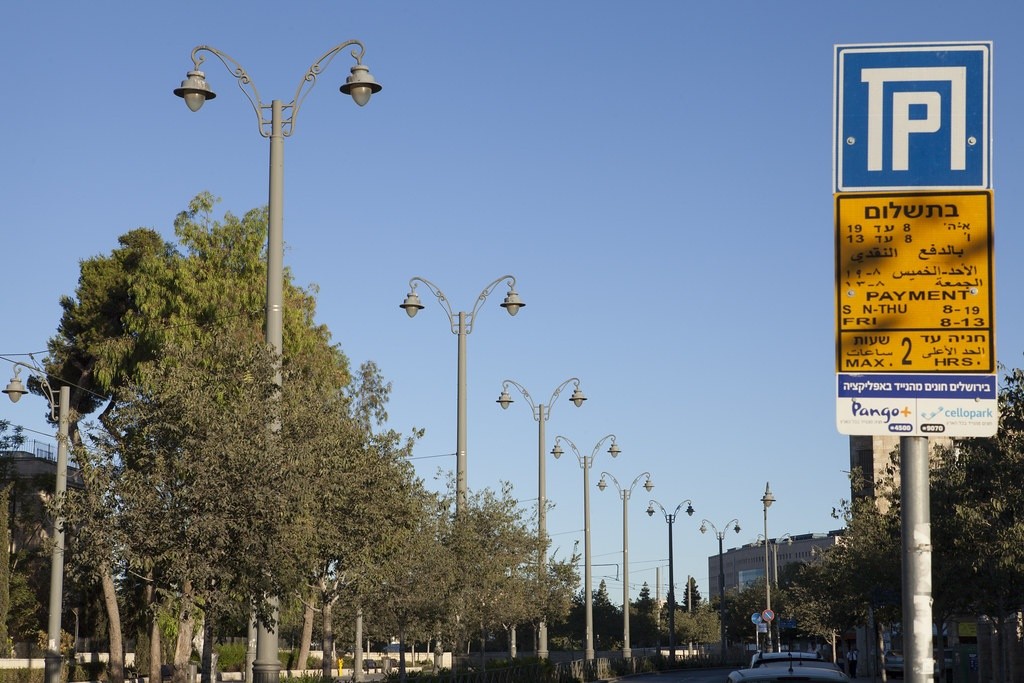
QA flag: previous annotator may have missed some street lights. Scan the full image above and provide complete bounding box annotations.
[698,518,742,666]
[646,497,696,669]
[550,434,621,681]
[594,471,655,675]
[496,374,588,683]
[0,361,73,683]
[170,39,386,683]
[396,275,528,683]
[760,482,776,653]
[755,533,794,652]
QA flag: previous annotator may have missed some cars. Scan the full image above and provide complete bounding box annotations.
[933,647,954,680]
[725,651,851,683]
[884,649,905,676]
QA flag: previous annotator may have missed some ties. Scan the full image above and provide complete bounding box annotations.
[852,652,853,660]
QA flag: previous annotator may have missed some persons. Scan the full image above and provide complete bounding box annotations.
[847,648,858,679]
